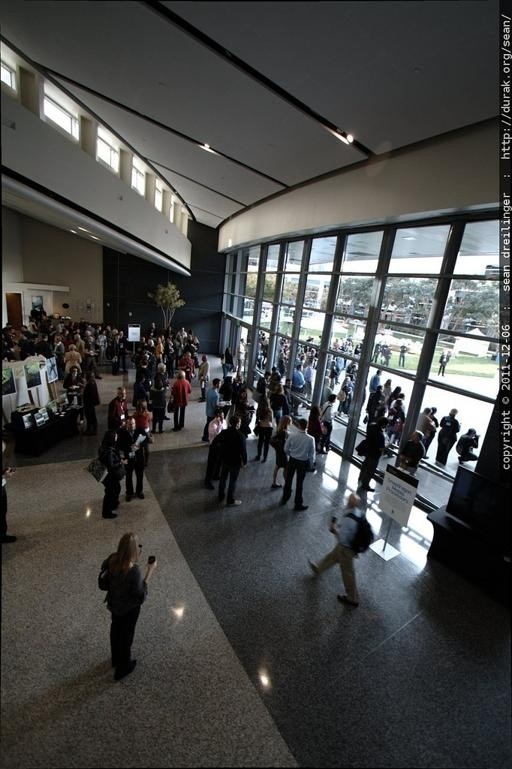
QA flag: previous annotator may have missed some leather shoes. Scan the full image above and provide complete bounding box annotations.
[114,660,136,681]
[337,593,360,605]
[295,505,309,511]
[271,484,282,488]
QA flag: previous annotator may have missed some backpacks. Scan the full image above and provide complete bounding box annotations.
[344,513,374,553]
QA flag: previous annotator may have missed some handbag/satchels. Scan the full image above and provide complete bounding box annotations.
[168,394,179,413]
[86,458,108,484]
[98,568,110,591]
[356,440,366,455]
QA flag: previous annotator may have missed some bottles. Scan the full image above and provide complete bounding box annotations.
[329,515,338,533]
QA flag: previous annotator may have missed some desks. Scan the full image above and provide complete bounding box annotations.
[11,404,84,458]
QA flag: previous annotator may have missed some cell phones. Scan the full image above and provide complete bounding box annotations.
[148,556,155,565]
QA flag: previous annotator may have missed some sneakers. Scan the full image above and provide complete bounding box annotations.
[136,493,144,499]
[104,513,118,518]
[125,494,134,502]
[230,500,242,506]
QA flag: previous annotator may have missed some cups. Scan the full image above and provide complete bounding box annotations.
[147,556,156,564]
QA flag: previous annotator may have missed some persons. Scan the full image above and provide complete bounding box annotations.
[438,349,450,377]
[399,429,426,475]
[98,530,157,680]
[270,414,293,489]
[457,427,481,465]
[434,408,461,467]
[254,407,274,464]
[306,490,373,608]
[203,409,229,491]
[120,416,150,503]
[0,441,21,542]
[208,414,248,508]
[1,307,439,455]
[86,428,125,520]
[276,418,316,511]
[355,415,389,493]
